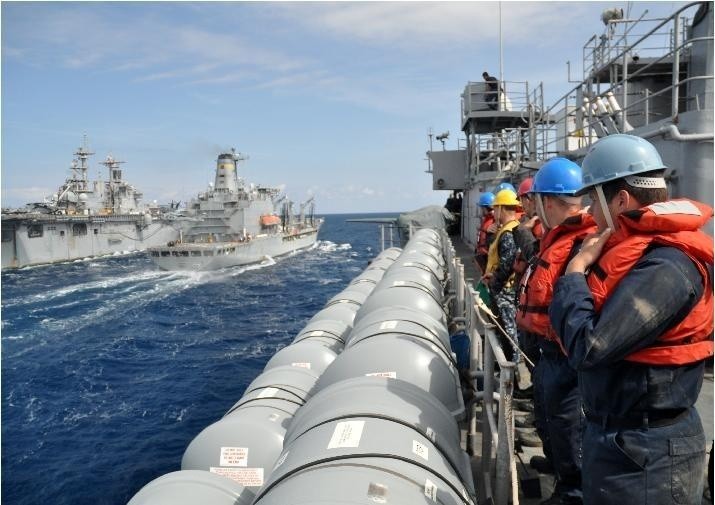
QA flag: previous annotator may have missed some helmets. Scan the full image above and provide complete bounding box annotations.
[574,132,668,198]
[476,177,532,208]
[526,156,584,197]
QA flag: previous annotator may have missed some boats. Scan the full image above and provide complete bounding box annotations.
[2,129,180,274]
[147,145,326,272]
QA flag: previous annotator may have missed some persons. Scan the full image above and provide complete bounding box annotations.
[512,176,550,447]
[515,155,600,504]
[482,72,506,111]
[552,133,714,505]
[493,181,519,198]
[475,189,497,273]
[483,190,523,383]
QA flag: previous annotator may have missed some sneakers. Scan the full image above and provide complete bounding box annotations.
[515,397,567,504]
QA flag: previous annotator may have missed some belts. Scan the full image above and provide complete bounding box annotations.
[578,405,694,432]
